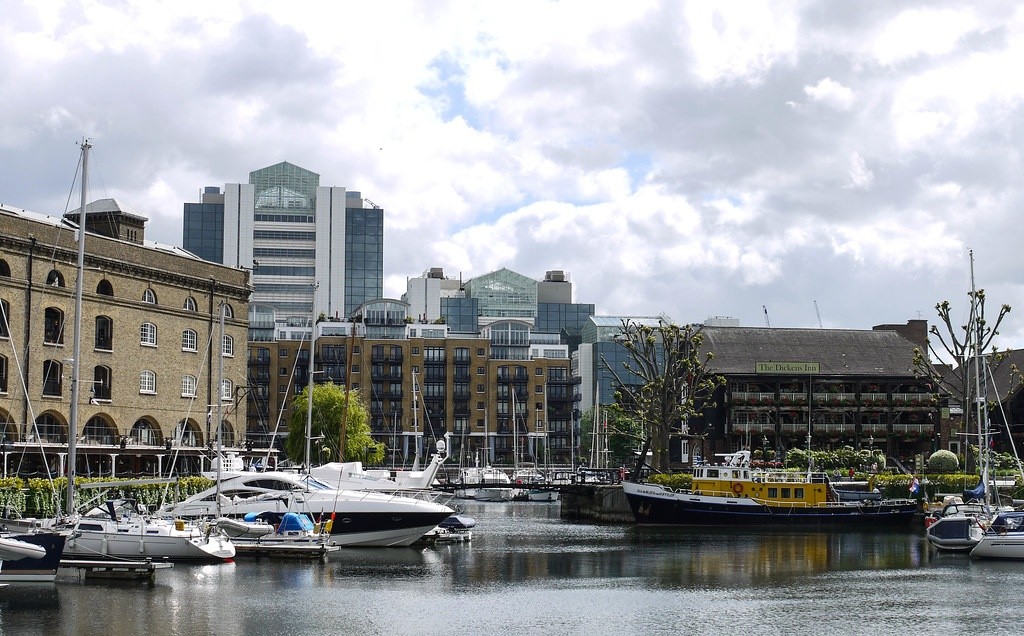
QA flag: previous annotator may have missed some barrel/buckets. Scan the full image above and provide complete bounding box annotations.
[175,522,184,530]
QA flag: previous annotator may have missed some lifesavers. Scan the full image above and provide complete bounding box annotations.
[733,482,743,493]
[924,516,938,527]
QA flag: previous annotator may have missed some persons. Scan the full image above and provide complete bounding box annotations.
[849,467,854,482]
[833,467,842,482]
[908,476,920,498]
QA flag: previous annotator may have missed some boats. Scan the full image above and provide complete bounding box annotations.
[621,352,918,525]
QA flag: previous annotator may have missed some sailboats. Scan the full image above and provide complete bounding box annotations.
[159,280,612,549]
[923,247,1024,561]
[0,131,344,586]
